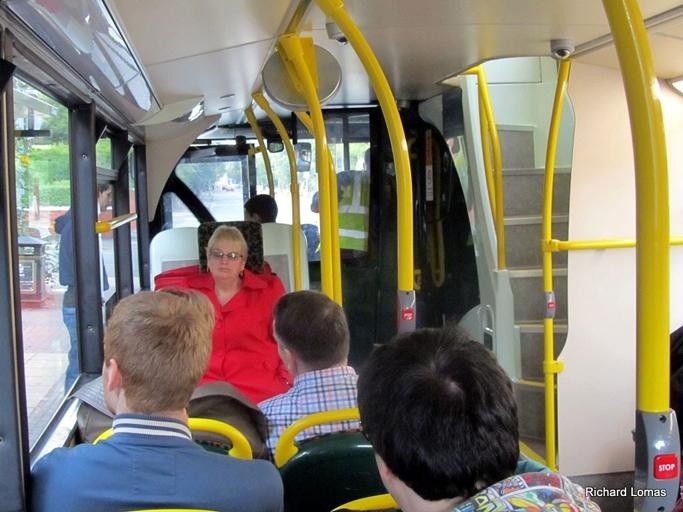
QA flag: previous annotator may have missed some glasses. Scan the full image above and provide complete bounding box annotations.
[209,249,242,261]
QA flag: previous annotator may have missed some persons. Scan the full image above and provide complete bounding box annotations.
[54,181,113,399]
[355,322,598,511]
[71,285,271,460]
[183,226,294,403]
[310,148,370,262]
[255,291,368,463]
[296,148,310,172]
[241,193,278,223]
[29,289,284,510]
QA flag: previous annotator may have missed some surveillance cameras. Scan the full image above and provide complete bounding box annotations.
[550,39,575,60]
[325,23,348,46]
